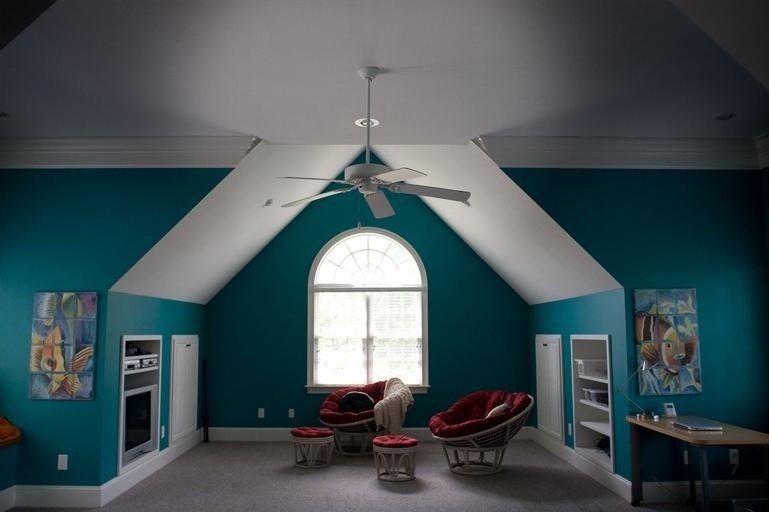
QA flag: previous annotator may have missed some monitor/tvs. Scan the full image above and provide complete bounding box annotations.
[122,384,160,466]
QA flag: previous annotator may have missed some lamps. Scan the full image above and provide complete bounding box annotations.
[269,67,472,222]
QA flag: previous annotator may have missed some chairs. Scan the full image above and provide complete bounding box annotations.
[319,378,415,457]
[428,389,534,476]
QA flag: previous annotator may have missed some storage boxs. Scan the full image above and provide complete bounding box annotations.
[574,358,607,376]
[580,388,608,404]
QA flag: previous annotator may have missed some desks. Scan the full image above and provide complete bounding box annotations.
[624,415,768,512]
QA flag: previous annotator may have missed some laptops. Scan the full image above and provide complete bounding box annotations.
[671,419,723,431]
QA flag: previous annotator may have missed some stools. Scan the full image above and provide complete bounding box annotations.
[371,435,419,483]
[290,425,335,470]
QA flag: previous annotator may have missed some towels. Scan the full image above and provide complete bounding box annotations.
[374,380,414,433]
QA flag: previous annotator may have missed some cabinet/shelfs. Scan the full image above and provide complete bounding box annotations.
[569,335,616,474]
[118,335,164,476]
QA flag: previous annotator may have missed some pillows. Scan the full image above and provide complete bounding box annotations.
[483,401,512,417]
[340,392,375,411]
